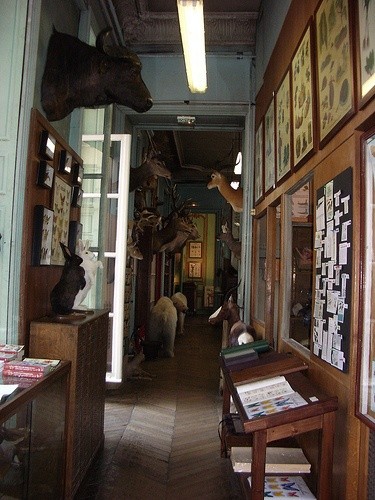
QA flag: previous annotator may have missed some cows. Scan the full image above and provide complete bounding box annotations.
[40,26,154,120]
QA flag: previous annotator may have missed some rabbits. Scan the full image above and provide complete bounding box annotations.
[50,238,103,316]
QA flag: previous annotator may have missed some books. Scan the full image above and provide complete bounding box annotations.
[0,375,39,389]
[220,339,318,500]
[0,344,60,379]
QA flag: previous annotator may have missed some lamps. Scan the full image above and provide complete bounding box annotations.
[175,0,208,92]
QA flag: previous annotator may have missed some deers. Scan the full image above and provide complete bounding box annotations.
[207,278,258,403]
[207,158,243,214]
[129,147,201,260]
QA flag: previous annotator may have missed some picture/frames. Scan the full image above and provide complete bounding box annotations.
[49,173,73,265]
[188,262,202,277]
[254,1,375,205]
[189,241,202,258]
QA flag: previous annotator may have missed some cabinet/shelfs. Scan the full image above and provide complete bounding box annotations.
[0,360,71,500]
[29,310,108,500]
[221,344,338,500]
